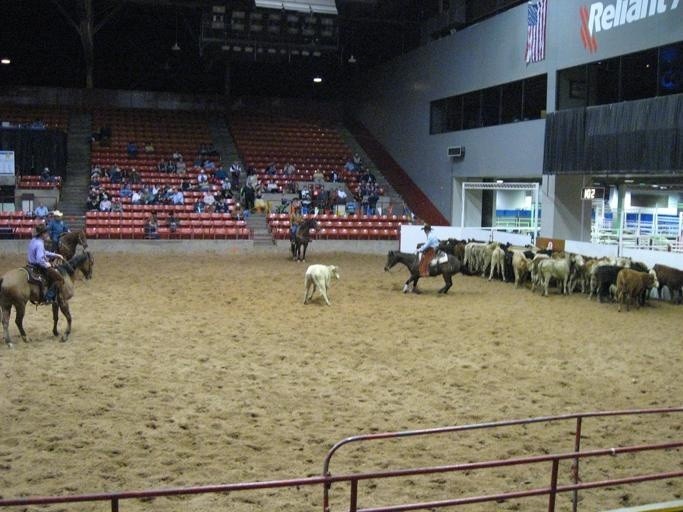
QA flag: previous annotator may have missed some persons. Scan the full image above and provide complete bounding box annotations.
[27,224,66,303]
[417,222,439,276]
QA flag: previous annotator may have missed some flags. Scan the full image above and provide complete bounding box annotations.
[524,0,548,63]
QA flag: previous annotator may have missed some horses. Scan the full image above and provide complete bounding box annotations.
[384,251,483,294]
[290,218,320,263]
[0,250,94,348]
[44,224,88,265]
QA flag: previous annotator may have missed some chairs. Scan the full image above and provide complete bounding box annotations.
[1,107,414,237]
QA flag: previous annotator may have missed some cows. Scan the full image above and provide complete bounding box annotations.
[439,239,683,312]
[304,264,340,307]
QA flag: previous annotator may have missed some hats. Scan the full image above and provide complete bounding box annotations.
[33,223,51,237]
[51,209,64,217]
[421,223,434,230]
